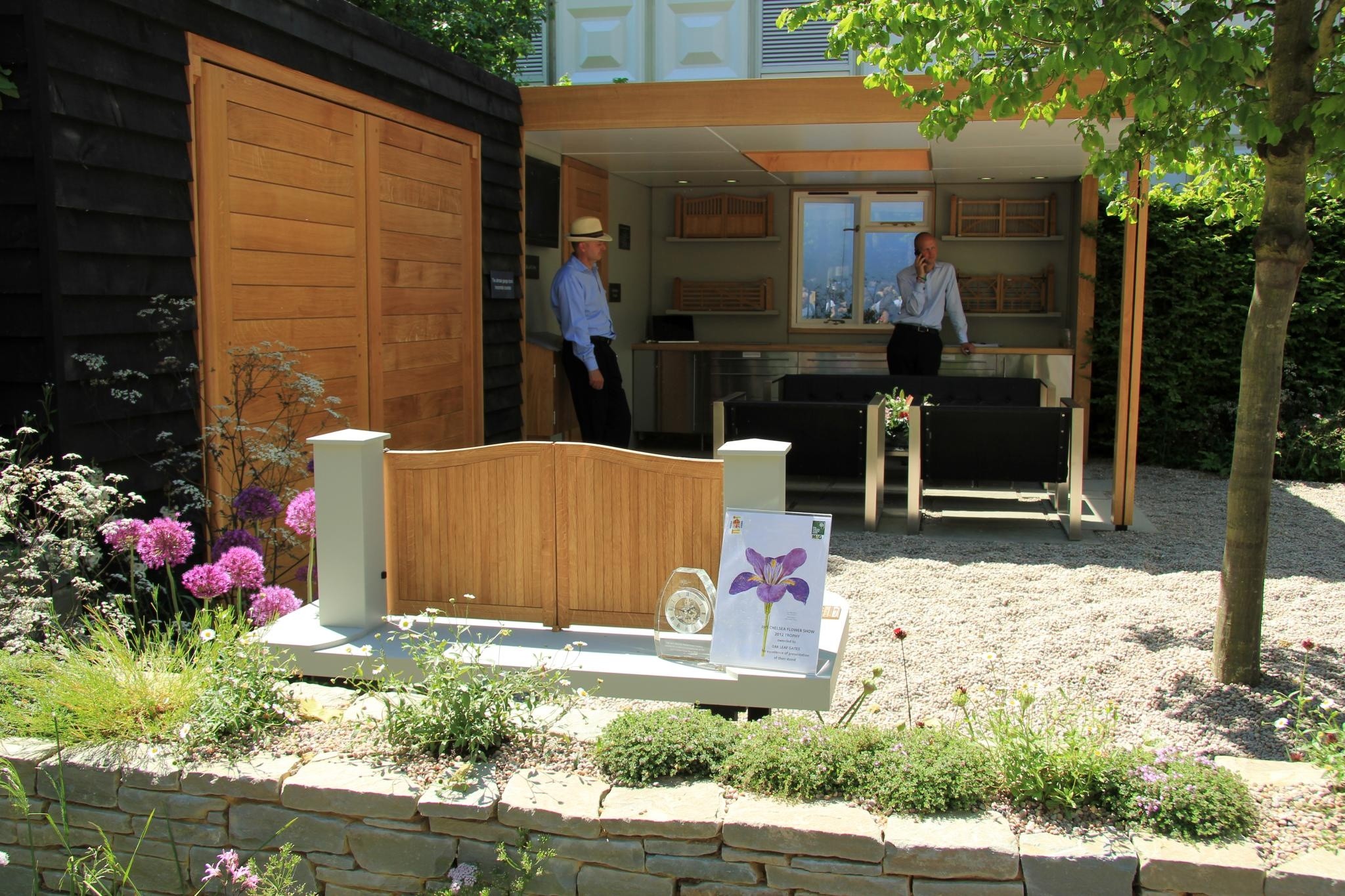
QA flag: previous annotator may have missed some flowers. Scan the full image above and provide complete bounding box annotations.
[872,383,941,448]
[728,545,811,657]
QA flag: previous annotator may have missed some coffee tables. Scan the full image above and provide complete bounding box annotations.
[884,443,908,458]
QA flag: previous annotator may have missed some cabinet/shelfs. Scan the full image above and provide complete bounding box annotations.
[938,354,1003,377]
[996,354,1073,408]
[663,234,780,315]
[631,349,696,435]
[798,351,891,375]
[939,235,1070,314]
[698,352,789,434]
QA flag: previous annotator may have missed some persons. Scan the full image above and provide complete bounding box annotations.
[552,215,634,452]
[885,232,976,375]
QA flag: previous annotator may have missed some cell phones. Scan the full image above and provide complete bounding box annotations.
[914,250,926,263]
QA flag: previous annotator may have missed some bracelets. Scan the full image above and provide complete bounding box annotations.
[916,276,927,282]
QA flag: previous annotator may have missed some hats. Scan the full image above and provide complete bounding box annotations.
[566,216,612,242]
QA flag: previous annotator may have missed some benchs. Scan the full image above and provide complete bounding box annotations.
[769,372,1057,491]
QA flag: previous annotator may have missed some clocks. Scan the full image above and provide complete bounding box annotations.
[652,566,718,665]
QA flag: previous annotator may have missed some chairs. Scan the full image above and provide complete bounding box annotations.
[708,389,886,530]
[905,394,1086,542]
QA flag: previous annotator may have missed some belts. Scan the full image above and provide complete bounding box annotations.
[563,334,614,345]
[894,323,939,334]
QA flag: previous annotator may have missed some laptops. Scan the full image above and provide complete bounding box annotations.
[650,313,701,345]
